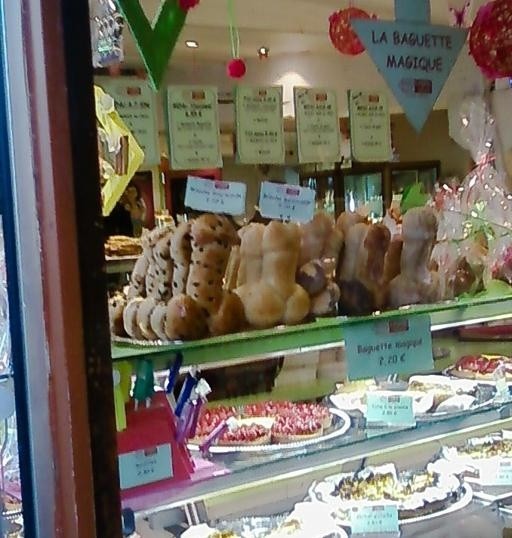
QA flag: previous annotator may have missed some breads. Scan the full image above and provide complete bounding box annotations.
[108,207,442,341]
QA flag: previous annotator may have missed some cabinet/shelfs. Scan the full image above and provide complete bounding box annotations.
[299,159,440,219]
[102,282,512,537]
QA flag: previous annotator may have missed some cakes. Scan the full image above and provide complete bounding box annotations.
[325,457,468,519]
[452,354,512,387]
[340,373,497,416]
[186,398,345,451]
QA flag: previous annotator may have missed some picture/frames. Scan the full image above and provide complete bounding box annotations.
[104,168,161,237]
